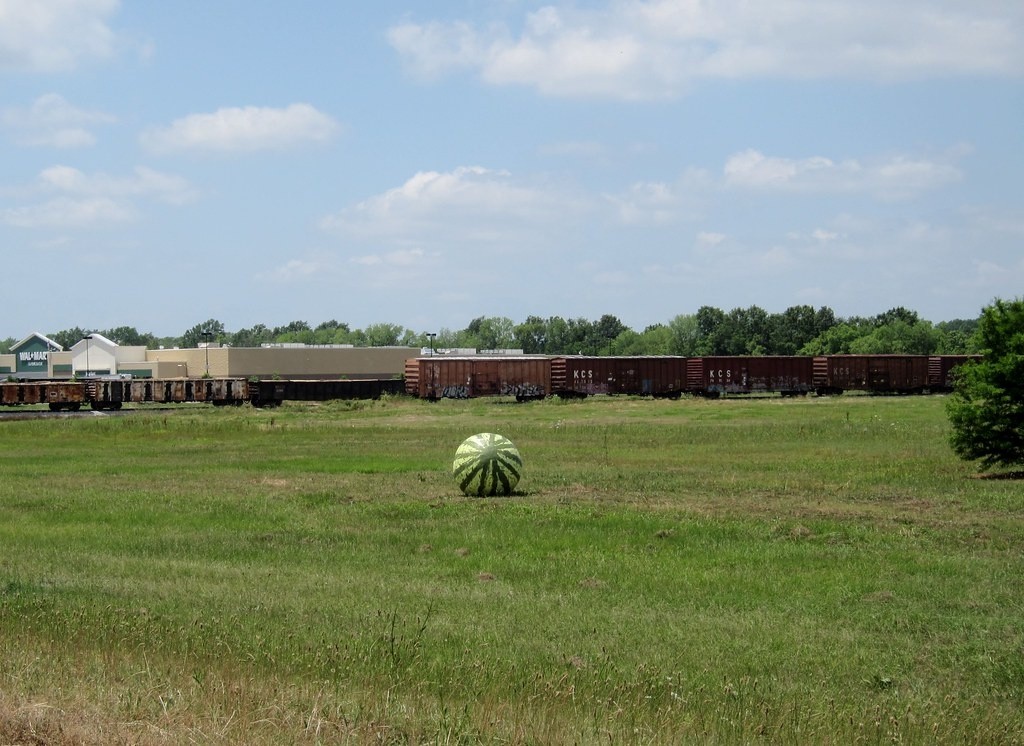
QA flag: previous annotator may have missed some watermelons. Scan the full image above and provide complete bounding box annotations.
[452,433,522,496]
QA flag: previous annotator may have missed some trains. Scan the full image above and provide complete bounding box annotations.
[404,353,983,402]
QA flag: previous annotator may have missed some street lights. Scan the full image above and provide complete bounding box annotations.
[201,332,213,374]
[83,336,93,377]
[426,333,436,357]
